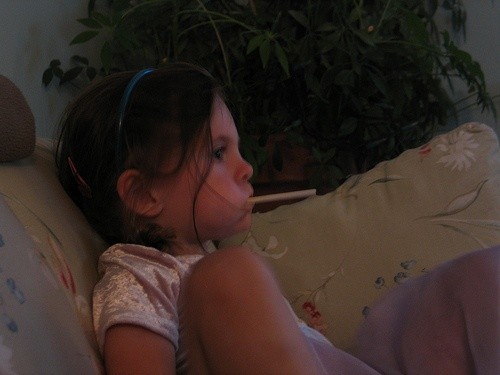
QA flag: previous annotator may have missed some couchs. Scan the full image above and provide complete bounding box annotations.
[0,138,215,375]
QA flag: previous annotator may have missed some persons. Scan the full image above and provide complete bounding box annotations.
[49,59,500,375]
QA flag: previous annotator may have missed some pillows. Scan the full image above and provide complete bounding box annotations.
[217,121,500,351]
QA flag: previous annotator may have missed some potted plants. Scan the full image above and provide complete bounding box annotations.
[41,0,497,185]
